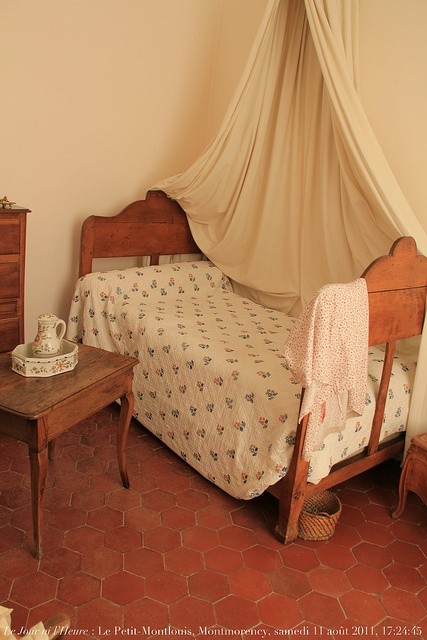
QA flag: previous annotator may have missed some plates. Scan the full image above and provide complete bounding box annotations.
[12,341,78,378]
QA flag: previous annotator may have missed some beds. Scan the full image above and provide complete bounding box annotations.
[62,186,427,548]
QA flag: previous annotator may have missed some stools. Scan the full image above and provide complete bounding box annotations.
[392,428,427,518]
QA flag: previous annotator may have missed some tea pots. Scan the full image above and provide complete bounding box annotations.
[32,313,66,359]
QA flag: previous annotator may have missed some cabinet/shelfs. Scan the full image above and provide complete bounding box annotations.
[0,205,33,353]
